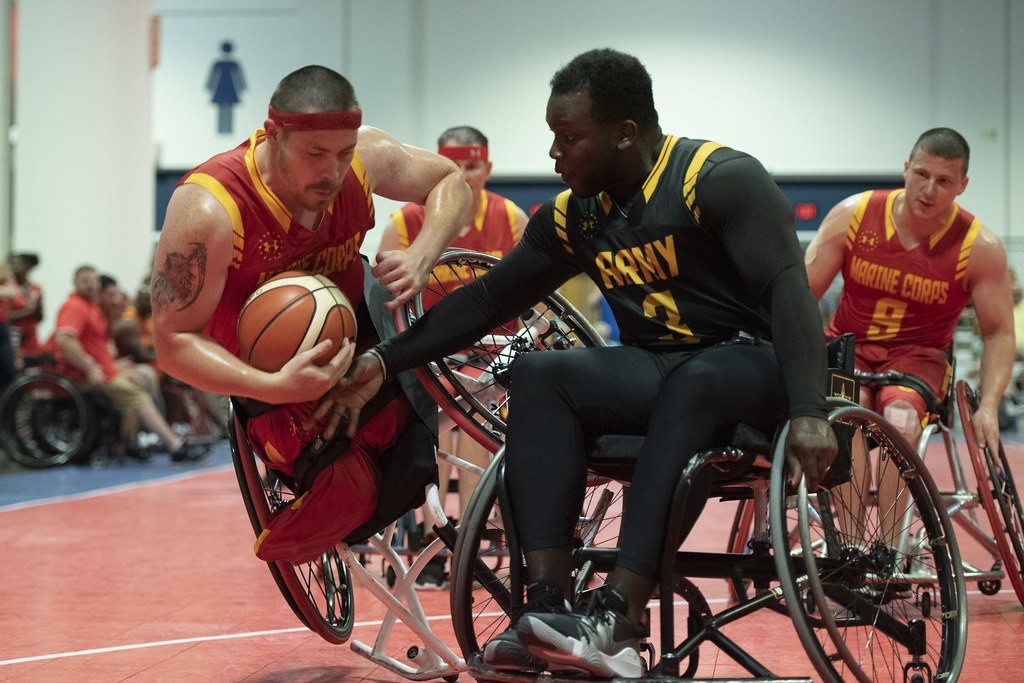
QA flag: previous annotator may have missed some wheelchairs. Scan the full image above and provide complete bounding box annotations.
[448,329,968,683]
[728,324,1024,616]
[221,246,607,683]
[0,352,117,469]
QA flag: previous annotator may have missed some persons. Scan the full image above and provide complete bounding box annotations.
[1,253,42,370]
[122,288,155,351]
[56,266,209,461]
[800,129,1015,605]
[39,276,166,416]
[376,127,531,592]
[306,49,836,683]
[151,66,473,567]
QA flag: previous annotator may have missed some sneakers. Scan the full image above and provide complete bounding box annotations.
[483,581,574,673]
[412,535,448,591]
[515,582,650,681]
[173,443,213,465]
[127,447,150,461]
[841,540,911,602]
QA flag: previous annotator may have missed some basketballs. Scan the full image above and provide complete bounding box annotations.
[237,270,357,373]
